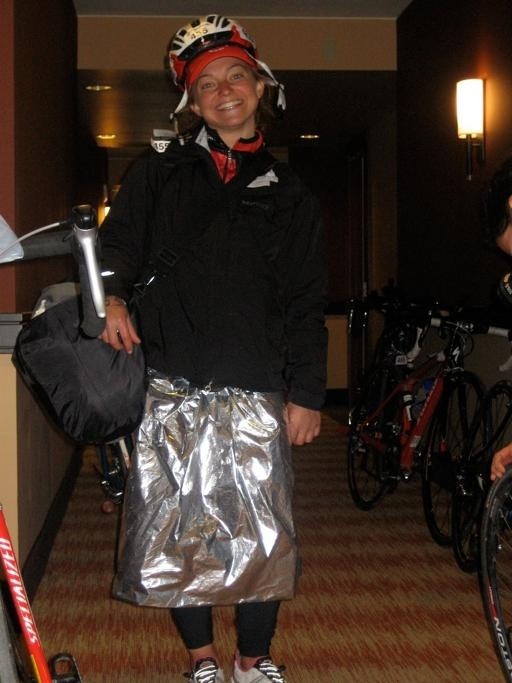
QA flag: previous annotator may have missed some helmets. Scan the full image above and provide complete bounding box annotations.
[167,14,258,86]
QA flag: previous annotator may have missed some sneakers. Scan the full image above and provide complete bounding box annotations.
[182,655,226,683]
[229,649,287,682]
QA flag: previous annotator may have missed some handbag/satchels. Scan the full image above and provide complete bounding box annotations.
[10,290,146,449]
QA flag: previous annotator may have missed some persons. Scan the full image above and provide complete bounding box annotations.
[78,12,331,681]
[480,179,510,485]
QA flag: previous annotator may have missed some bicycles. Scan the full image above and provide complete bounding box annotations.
[336,280,512,681]
[12,205,135,517]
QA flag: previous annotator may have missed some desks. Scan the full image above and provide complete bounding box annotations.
[325,313,351,390]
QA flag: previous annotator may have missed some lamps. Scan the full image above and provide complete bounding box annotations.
[456,79,485,179]
